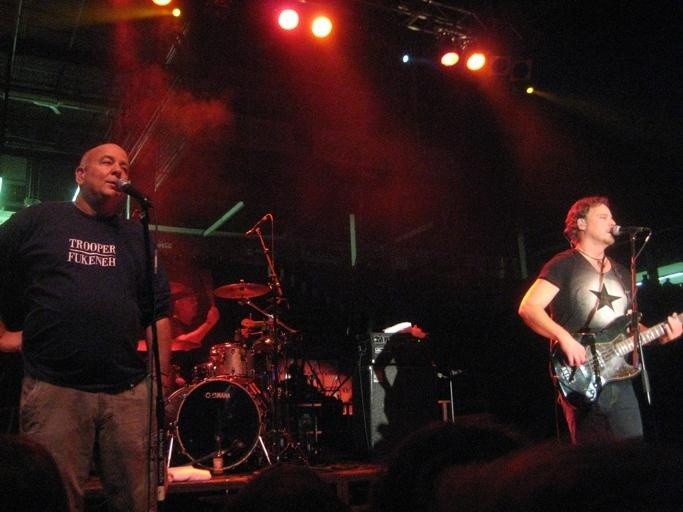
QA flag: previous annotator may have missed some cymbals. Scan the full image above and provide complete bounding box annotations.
[213,282,271,298]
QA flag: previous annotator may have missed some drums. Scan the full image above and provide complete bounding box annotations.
[209,342,255,380]
[169,383,198,413]
[191,362,210,383]
[175,375,269,474]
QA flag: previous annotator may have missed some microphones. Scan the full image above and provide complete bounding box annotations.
[244,215,268,238]
[117,177,149,207]
[611,225,647,236]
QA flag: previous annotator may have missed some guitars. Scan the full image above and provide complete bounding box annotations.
[549,308,683,406]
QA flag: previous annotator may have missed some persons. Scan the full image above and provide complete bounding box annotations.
[0,143,221,512]
[373,313,434,434]
[0,416,683,512]
[515,194,683,444]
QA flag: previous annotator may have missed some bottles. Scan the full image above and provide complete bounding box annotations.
[211,433,224,475]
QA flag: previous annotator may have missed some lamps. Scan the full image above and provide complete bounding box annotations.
[435,30,489,72]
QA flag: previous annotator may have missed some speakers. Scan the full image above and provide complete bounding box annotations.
[348,365,436,463]
